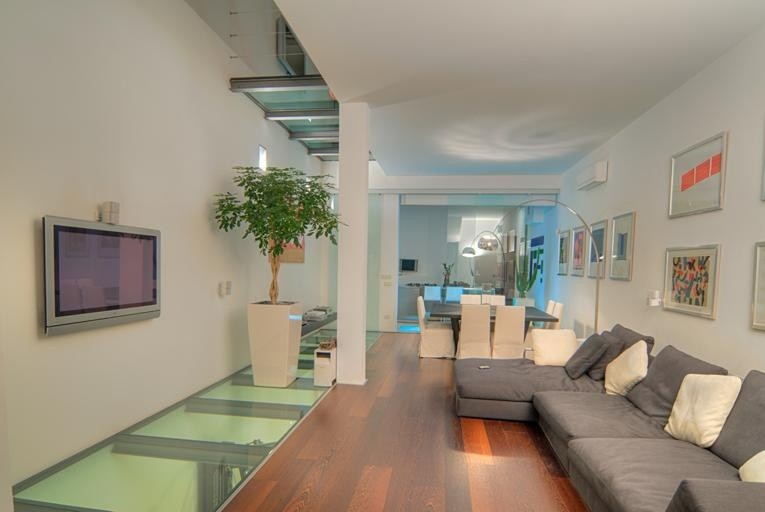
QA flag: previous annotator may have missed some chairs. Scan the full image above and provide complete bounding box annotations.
[415,292,564,360]
[425,286,463,304]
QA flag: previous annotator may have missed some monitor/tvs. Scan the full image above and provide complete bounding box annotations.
[399,258,418,272]
[43,215,161,337]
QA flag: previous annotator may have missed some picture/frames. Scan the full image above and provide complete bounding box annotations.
[666,132,727,219]
[664,244,721,321]
[750,241,765,331]
[557,211,637,281]
[760,126,765,200]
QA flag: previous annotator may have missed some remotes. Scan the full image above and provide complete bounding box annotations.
[480,366,490,368]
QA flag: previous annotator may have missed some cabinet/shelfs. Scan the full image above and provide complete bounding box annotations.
[313,347,337,387]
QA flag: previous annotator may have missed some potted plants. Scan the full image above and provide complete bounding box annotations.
[211,164,350,388]
[513,223,540,306]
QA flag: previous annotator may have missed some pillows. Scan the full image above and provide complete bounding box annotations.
[564,324,765,482]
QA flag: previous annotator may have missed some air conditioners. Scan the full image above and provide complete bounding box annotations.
[575,161,608,190]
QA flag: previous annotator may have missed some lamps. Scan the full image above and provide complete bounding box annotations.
[461,230,509,295]
[478,198,599,332]
